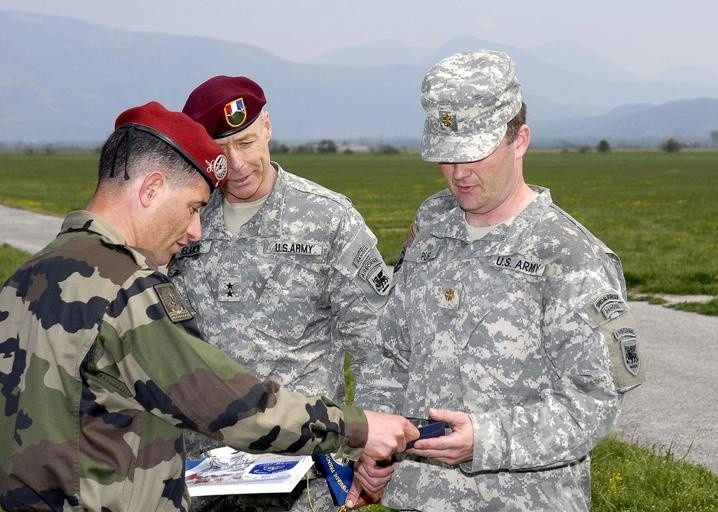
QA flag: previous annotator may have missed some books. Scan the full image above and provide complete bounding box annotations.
[185,451,314,498]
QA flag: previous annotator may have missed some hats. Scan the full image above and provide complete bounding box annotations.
[114,101,228,195]
[181,74,266,139]
[420,48,523,164]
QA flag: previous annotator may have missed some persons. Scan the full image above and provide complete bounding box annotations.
[353,49,644,512]
[1,102,420,512]
[163,75,393,511]
